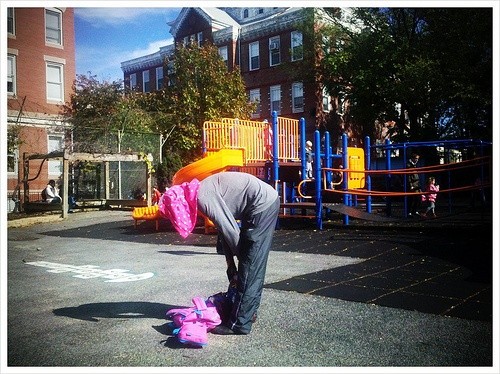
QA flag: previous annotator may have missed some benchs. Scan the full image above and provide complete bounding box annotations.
[74,199,147,212]
[28,203,63,217]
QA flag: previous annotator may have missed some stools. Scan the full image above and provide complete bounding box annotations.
[132,206,160,230]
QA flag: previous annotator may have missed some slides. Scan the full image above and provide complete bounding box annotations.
[133,148,244,229]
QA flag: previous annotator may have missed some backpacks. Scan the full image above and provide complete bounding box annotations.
[41,188,52,199]
[165,299,222,349]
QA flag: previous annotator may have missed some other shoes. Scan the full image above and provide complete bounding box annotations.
[210,324,243,335]
[221,308,257,323]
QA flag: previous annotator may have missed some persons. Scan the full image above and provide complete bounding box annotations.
[158,172,280,336]
[305,140,314,180]
[152,185,162,205]
[44,180,63,213]
[406,153,440,217]
[263,118,275,160]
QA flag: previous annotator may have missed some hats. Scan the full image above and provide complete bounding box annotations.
[158,178,199,239]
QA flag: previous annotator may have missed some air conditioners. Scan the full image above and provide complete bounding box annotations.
[269,43,278,51]
